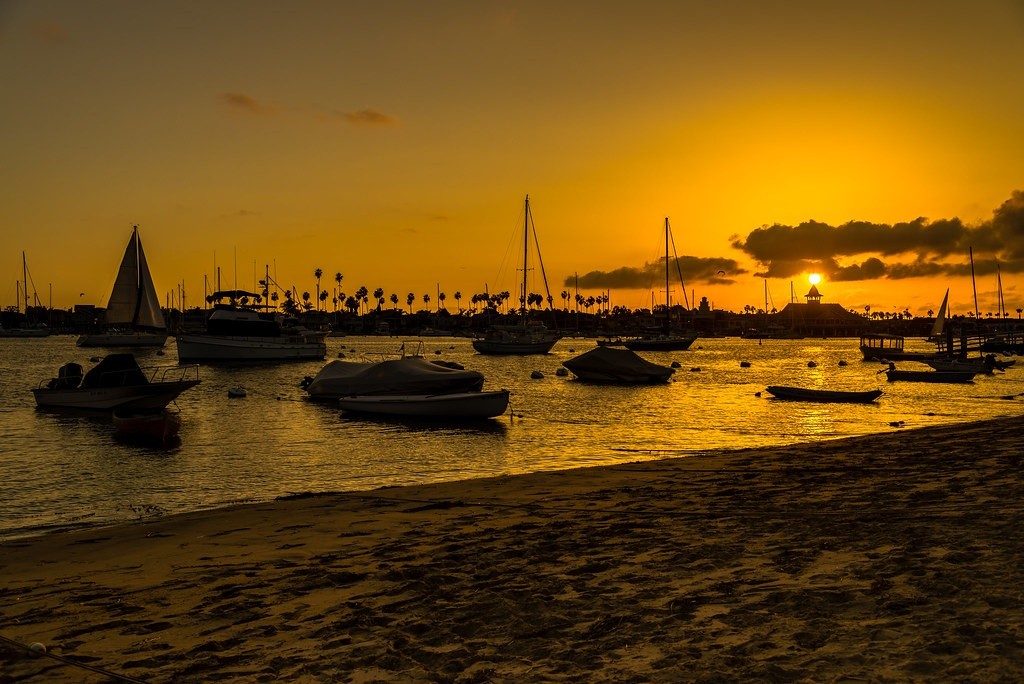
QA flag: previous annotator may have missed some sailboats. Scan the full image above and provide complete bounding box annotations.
[473,193,561,354]
[0,252,49,337]
[741,281,806,339]
[76,226,168,347]
[926,288,951,342]
[596,218,698,349]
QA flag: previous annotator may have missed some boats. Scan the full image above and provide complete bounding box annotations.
[564,347,676,383]
[34,355,202,416]
[922,354,995,373]
[308,339,484,400]
[176,304,326,364]
[996,361,1016,368]
[886,363,976,382]
[340,390,509,422]
[765,384,884,402]
[114,407,180,443]
[860,334,967,360]
